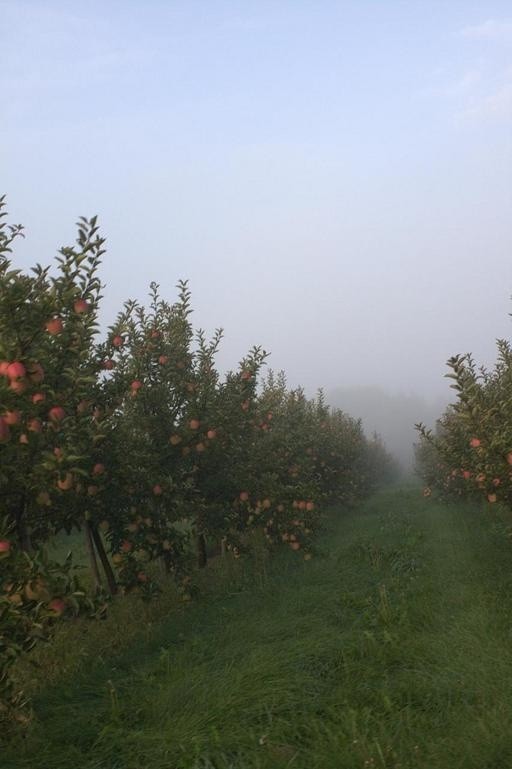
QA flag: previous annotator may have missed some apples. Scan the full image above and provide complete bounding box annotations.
[0,300,314,614]
[451,437,512,504]
